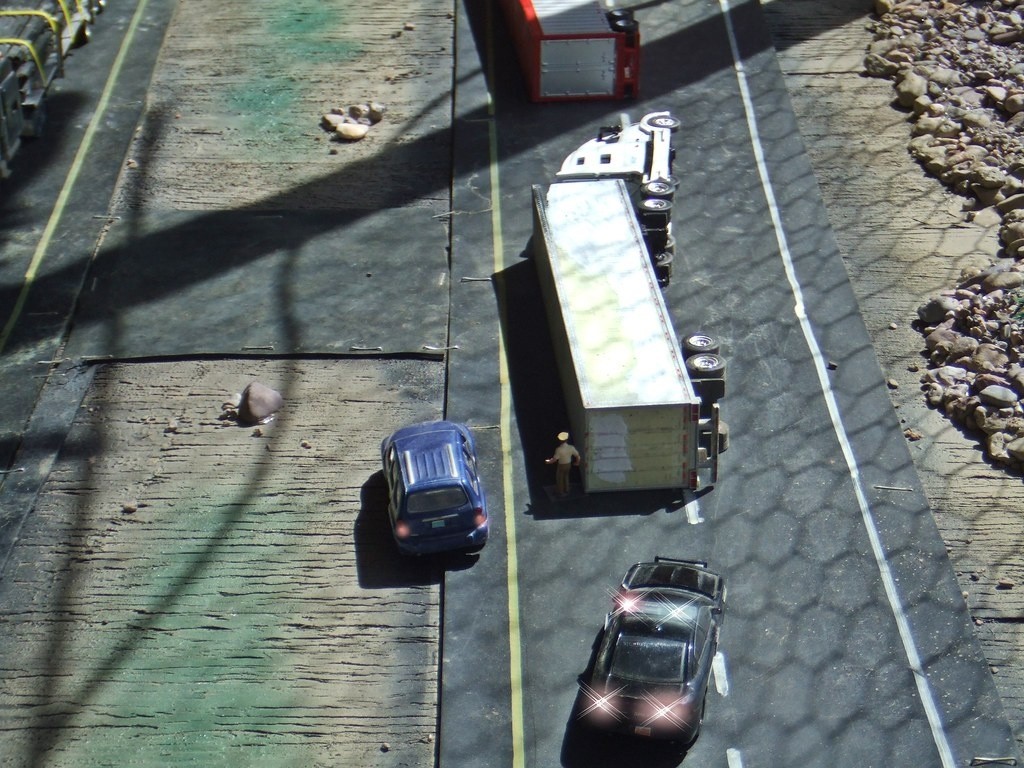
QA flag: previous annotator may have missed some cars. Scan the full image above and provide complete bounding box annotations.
[576,556,726,754]
[376,416,489,559]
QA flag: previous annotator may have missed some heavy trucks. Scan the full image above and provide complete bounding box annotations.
[524,107,730,506]
